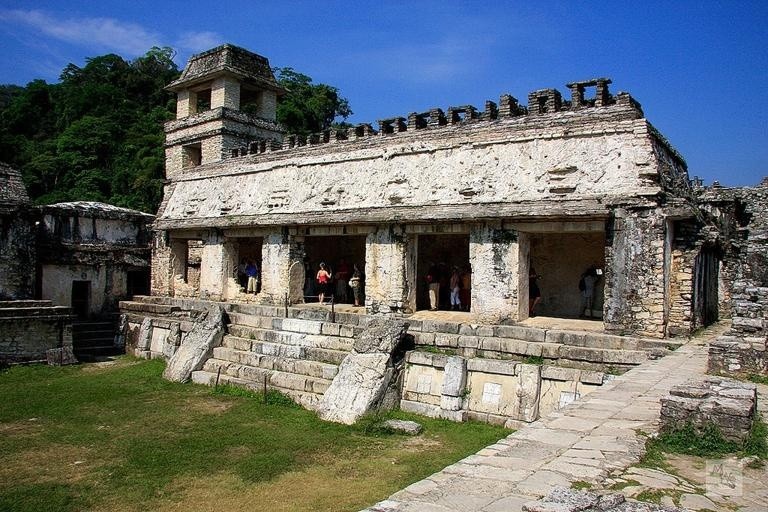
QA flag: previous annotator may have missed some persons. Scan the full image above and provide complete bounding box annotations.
[579,268,600,317]
[349,263,362,307]
[245,255,260,295]
[240,256,247,289]
[335,257,348,304]
[529,257,541,317]
[449,267,463,311]
[316,262,333,304]
[424,259,442,311]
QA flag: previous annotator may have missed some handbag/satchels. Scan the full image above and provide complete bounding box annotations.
[329,272,332,273]
